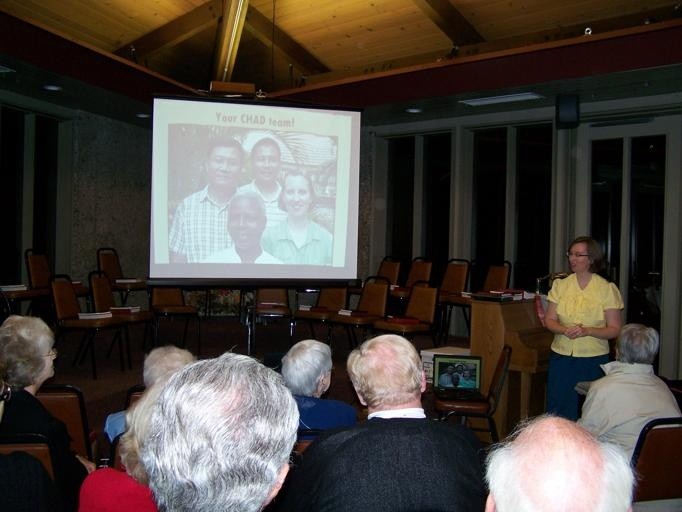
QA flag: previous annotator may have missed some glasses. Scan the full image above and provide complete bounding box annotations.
[38,348,57,358]
[0,386,12,402]
[566,251,592,257]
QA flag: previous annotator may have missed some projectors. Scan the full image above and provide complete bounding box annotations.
[263,352,286,373]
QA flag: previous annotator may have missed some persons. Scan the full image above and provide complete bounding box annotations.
[168,137,246,263]
[544,237,625,422]
[263,174,333,265]
[210,193,282,263]
[241,137,287,246]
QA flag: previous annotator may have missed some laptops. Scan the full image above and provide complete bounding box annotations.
[433,354,487,402]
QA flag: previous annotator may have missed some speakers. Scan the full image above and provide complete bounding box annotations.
[555,94,579,129]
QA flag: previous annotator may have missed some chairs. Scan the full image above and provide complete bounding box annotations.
[1,247,514,379]
[289,433,315,462]
[631,417,682,503]
[3,383,143,486]
[436,346,511,445]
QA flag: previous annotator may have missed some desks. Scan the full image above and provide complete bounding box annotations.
[472,300,551,441]
